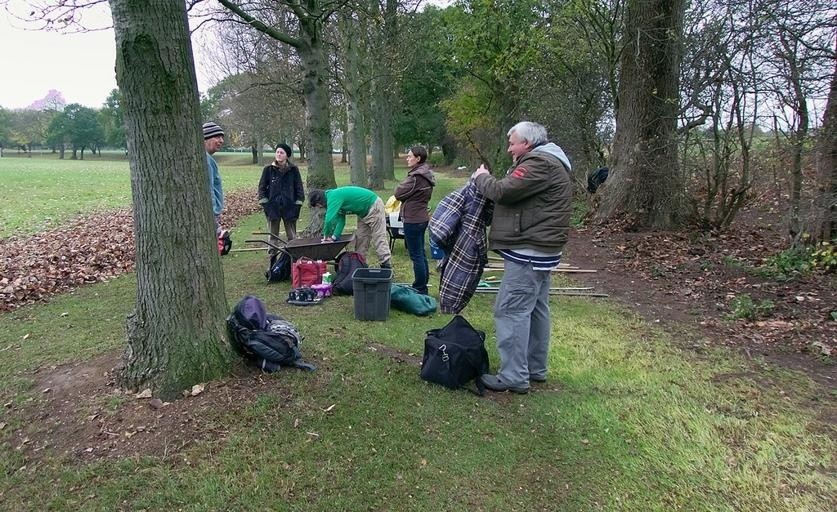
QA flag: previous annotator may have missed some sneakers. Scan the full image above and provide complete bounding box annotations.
[481,371,530,395]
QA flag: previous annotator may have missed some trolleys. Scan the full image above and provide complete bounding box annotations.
[244,226,359,276]
[383,214,408,255]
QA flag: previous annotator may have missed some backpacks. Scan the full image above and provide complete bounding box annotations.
[332,250,366,297]
[225,295,316,374]
[263,251,291,283]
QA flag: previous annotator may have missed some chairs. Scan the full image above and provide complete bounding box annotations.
[386,215,407,253]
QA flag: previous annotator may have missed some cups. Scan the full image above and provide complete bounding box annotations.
[288,287,317,301]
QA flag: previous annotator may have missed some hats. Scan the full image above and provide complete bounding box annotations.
[202,121,224,139]
[307,190,322,208]
[529,368,547,383]
[275,144,292,157]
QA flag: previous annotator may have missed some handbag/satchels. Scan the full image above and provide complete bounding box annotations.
[289,257,333,306]
[420,314,489,391]
[218,229,232,256]
[587,166,609,193]
[390,283,437,317]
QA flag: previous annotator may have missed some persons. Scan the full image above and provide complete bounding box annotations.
[309,184,390,269]
[473,122,574,392]
[202,122,224,223]
[394,146,435,292]
[260,144,303,269]
